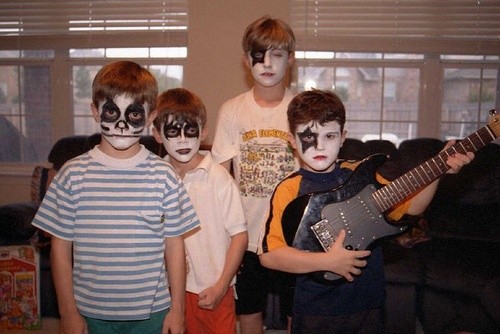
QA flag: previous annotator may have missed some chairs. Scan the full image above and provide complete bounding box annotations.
[336,137,499,334]
[49,133,168,168]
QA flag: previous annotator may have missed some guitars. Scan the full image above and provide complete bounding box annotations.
[280,109,500,281]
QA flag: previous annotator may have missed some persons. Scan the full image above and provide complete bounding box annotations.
[31,61,201,334]
[211,15,305,334]
[256,88,475,334]
[153,88,248,334]
